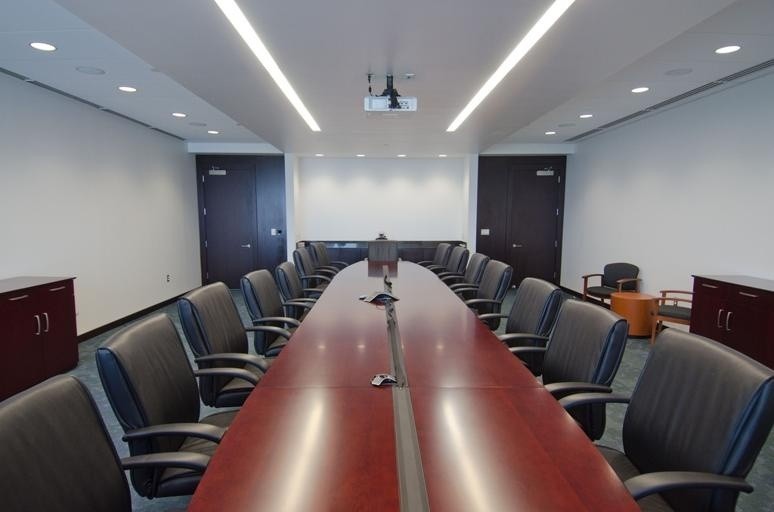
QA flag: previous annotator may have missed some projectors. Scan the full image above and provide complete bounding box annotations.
[364,96,418,112]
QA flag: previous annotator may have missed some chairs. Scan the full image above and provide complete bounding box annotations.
[582,263,643,303]
[177,282,291,409]
[310,242,349,273]
[293,247,336,298]
[508,299,630,393]
[652,290,694,347]
[275,262,325,328]
[240,269,313,357]
[453,260,513,330]
[558,328,774,512]
[417,243,452,269]
[1,374,132,512]
[429,246,470,277]
[477,277,563,342]
[440,253,490,290]
[95,313,260,501]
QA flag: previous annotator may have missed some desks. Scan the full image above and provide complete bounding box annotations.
[610,293,660,336]
[188,256,642,511]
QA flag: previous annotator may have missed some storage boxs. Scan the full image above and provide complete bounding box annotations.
[689,274,774,371]
[0,276,79,403]
